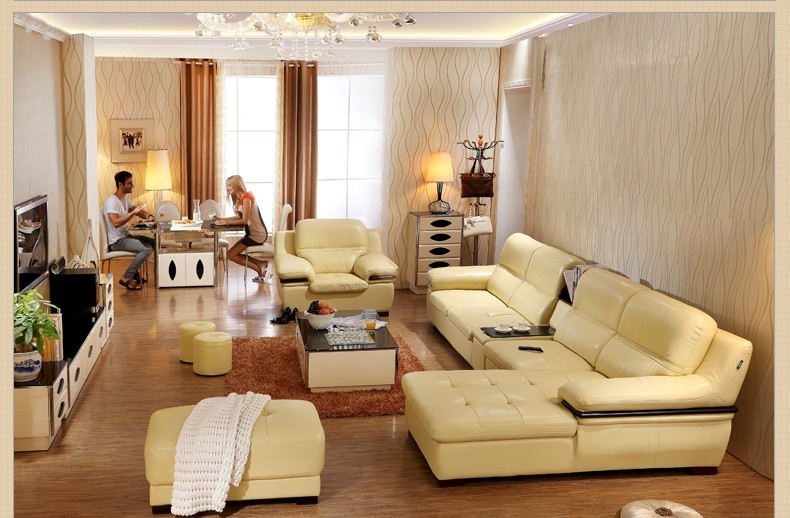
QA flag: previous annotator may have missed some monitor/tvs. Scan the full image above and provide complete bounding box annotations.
[14,194,49,299]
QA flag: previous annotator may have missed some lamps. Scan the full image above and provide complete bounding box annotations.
[424,151,454,216]
[186,12,416,70]
[145,150,172,209]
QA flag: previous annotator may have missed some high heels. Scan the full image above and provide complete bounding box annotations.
[270,306,299,324]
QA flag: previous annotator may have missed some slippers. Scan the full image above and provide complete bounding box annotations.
[119,280,142,290]
[133,273,147,283]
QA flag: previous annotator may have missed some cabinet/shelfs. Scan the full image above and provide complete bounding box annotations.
[406,212,464,295]
[13,273,113,451]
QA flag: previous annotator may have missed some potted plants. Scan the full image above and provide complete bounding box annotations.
[14,289,61,383]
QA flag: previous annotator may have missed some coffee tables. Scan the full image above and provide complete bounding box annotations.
[293,309,399,392]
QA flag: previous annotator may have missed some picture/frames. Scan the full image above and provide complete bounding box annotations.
[111,120,156,164]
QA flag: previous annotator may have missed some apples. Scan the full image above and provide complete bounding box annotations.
[319,300,332,315]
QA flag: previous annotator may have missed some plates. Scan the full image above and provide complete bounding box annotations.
[494,327,512,333]
[512,326,531,332]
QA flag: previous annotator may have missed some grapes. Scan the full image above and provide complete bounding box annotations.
[308,301,320,314]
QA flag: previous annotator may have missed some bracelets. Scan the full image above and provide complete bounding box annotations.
[148,214,154,219]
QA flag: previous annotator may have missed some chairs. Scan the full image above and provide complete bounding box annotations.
[100,208,148,286]
[156,201,180,223]
[200,199,229,276]
[244,203,293,286]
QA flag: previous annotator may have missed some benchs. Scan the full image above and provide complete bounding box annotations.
[142,399,325,514]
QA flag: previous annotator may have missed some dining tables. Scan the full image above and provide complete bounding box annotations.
[127,220,246,288]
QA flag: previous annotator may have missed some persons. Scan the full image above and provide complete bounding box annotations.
[213,175,268,282]
[103,171,157,290]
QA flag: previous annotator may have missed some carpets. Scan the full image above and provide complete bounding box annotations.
[227,334,427,417]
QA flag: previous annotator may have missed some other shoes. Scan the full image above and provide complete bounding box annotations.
[252,277,263,281]
[262,260,269,277]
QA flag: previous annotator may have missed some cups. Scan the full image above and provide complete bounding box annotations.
[500,323,510,331]
[517,322,527,329]
[365,310,377,334]
[208,211,216,222]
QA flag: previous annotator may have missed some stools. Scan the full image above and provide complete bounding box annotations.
[191,332,233,377]
[620,500,705,518]
[178,322,218,364]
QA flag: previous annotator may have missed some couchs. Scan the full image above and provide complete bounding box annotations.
[271,219,399,319]
[402,232,753,485]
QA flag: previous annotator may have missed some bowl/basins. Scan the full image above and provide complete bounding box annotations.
[145,223,154,227]
[304,308,337,330]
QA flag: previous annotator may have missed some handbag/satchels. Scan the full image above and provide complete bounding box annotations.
[459,158,496,198]
[462,203,493,237]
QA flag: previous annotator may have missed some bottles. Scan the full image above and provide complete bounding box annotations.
[193,199,202,221]
